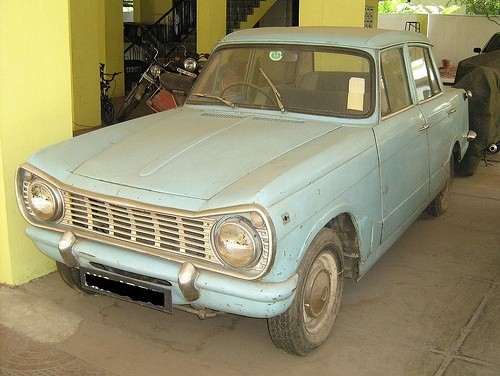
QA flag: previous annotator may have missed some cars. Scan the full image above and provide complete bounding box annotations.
[474,32,499,54]
[15,25,477,359]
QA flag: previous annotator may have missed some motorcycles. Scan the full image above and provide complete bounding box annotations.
[117,35,202,123]
[167,44,211,79]
[449,50,499,177]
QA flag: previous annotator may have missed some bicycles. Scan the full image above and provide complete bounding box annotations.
[99,64,123,124]
